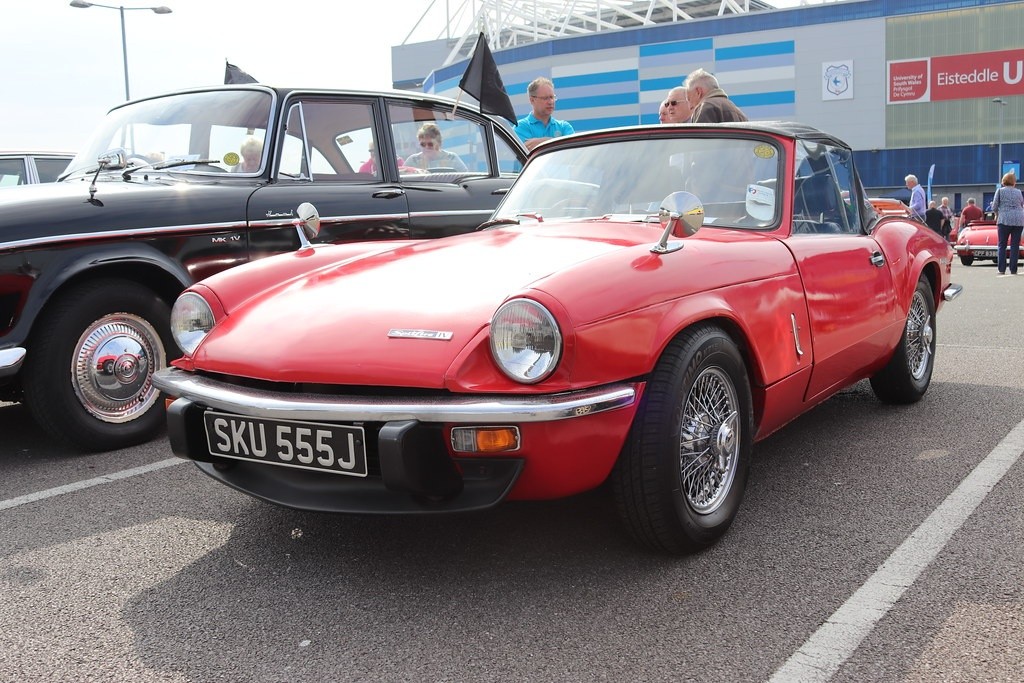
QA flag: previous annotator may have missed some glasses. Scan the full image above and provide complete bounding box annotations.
[665,100,686,107]
[531,95,557,101]
[419,141,438,148]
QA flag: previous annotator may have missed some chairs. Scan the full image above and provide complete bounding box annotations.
[753,176,841,232]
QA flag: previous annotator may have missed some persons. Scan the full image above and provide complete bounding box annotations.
[937,197,955,240]
[986,201,993,212]
[231,137,263,172]
[958,198,983,234]
[402,124,469,172]
[359,139,403,174]
[510,76,576,172]
[925,201,945,235]
[990,172,1024,274]
[905,174,926,224]
[660,69,756,203]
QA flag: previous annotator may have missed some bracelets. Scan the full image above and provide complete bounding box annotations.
[994,214,998,215]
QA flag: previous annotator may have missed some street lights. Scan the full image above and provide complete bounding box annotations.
[992,98,1007,189]
[70,0,173,154]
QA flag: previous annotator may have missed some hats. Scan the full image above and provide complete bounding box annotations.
[967,198,975,203]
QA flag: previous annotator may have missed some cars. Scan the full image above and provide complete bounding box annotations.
[96,354,140,375]
[949,217,959,245]
[0,84,600,452]
[0,148,78,188]
[152,119,961,553]
[840,190,912,218]
[954,211,1024,266]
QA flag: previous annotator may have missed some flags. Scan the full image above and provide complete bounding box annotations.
[224,58,259,85]
[459,32,518,127]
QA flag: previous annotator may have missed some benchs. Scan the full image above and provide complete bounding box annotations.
[367,171,488,189]
